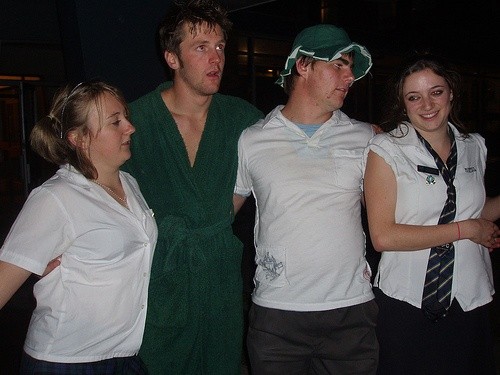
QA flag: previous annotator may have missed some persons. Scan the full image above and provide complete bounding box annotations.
[37,0,270,375]
[364,51,500,375]
[0,76,159,375]
[231,25,500,375]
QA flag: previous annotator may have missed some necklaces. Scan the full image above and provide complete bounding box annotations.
[88,178,126,202]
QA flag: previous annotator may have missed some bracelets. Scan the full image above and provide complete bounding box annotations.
[456,222,460,241]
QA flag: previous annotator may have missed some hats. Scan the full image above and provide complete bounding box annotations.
[273,24,372,90]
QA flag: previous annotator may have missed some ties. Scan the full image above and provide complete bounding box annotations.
[414,124,457,311]
